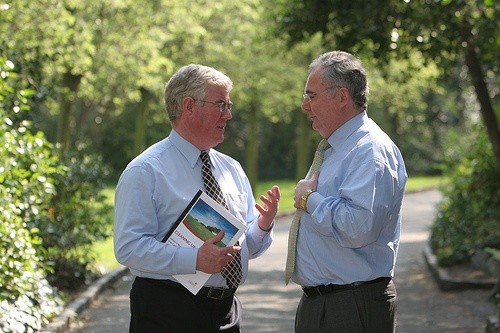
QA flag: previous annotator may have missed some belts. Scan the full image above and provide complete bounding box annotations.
[159,280,235,300]
[300,277,391,298]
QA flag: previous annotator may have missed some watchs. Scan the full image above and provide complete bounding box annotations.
[300,190,315,212]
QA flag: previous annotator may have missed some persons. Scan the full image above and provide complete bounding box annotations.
[113,63,280,332]
[285,51,408,333]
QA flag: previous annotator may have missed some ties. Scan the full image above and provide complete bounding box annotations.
[200,151,243,291]
[285,137,331,287]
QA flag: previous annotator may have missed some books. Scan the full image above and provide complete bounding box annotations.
[160,189,248,296]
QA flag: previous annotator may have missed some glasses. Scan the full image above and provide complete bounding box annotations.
[302,85,342,102]
[195,99,233,113]
